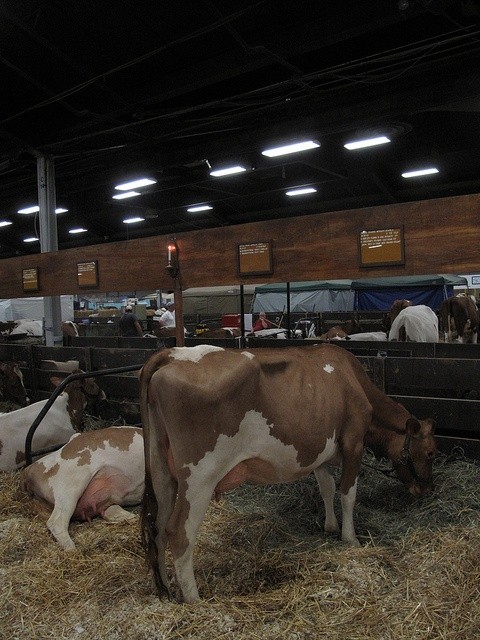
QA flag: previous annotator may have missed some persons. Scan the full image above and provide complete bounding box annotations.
[155,300,189,336]
[119,305,143,337]
[253,311,272,332]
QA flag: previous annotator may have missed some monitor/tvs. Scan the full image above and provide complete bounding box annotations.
[356,223,405,268]
[235,239,274,275]
[21,266,39,291]
[76,259,99,288]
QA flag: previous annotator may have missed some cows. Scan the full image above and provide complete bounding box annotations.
[320,319,387,341]
[138,342,438,603]
[382,299,414,342]
[20,425,145,552]
[188,326,242,348]
[440,293,480,344]
[9,320,79,337]
[23,360,86,392]
[0,369,106,472]
[0,360,30,406]
[0,319,39,335]
[142,325,196,338]
[388,302,439,341]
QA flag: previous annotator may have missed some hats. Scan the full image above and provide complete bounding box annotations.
[166,302,175,308]
[125,306,132,311]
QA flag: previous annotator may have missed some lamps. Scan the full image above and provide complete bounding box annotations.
[164,238,178,268]
